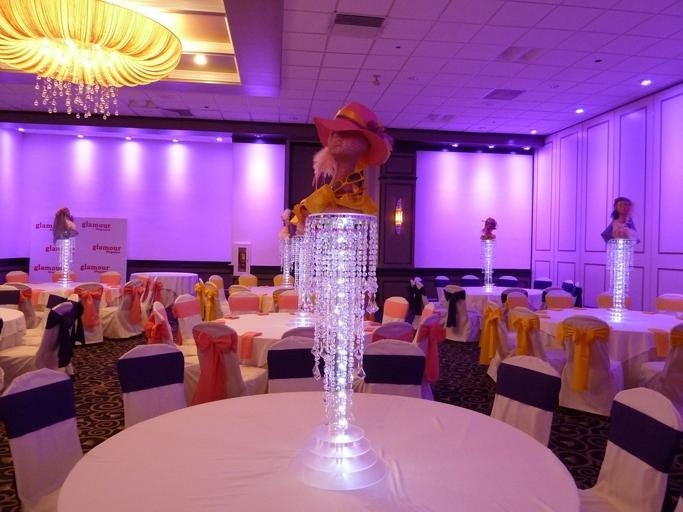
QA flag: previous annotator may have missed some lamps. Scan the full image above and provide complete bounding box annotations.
[391,197,404,239]
[1,1,183,129]
[277,99,398,491]
[476,215,498,294]
[597,196,642,323]
[51,206,79,289]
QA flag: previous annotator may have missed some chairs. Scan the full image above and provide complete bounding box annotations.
[489,355,561,451]
[188,322,268,407]
[0,261,313,367]
[1,301,81,382]
[264,336,323,391]
[576,386,682,512]
[359,339,426,400]
[118,344,186,430]
[0,367,83,512]
[364,259,683,384]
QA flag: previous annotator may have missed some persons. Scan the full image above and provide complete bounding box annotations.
[480,217,496,240]
[279,208,297,239]
[291,101,389,215]
[601,197,640,241]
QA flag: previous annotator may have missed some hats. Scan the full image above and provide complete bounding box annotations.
[314,102,393,165]
